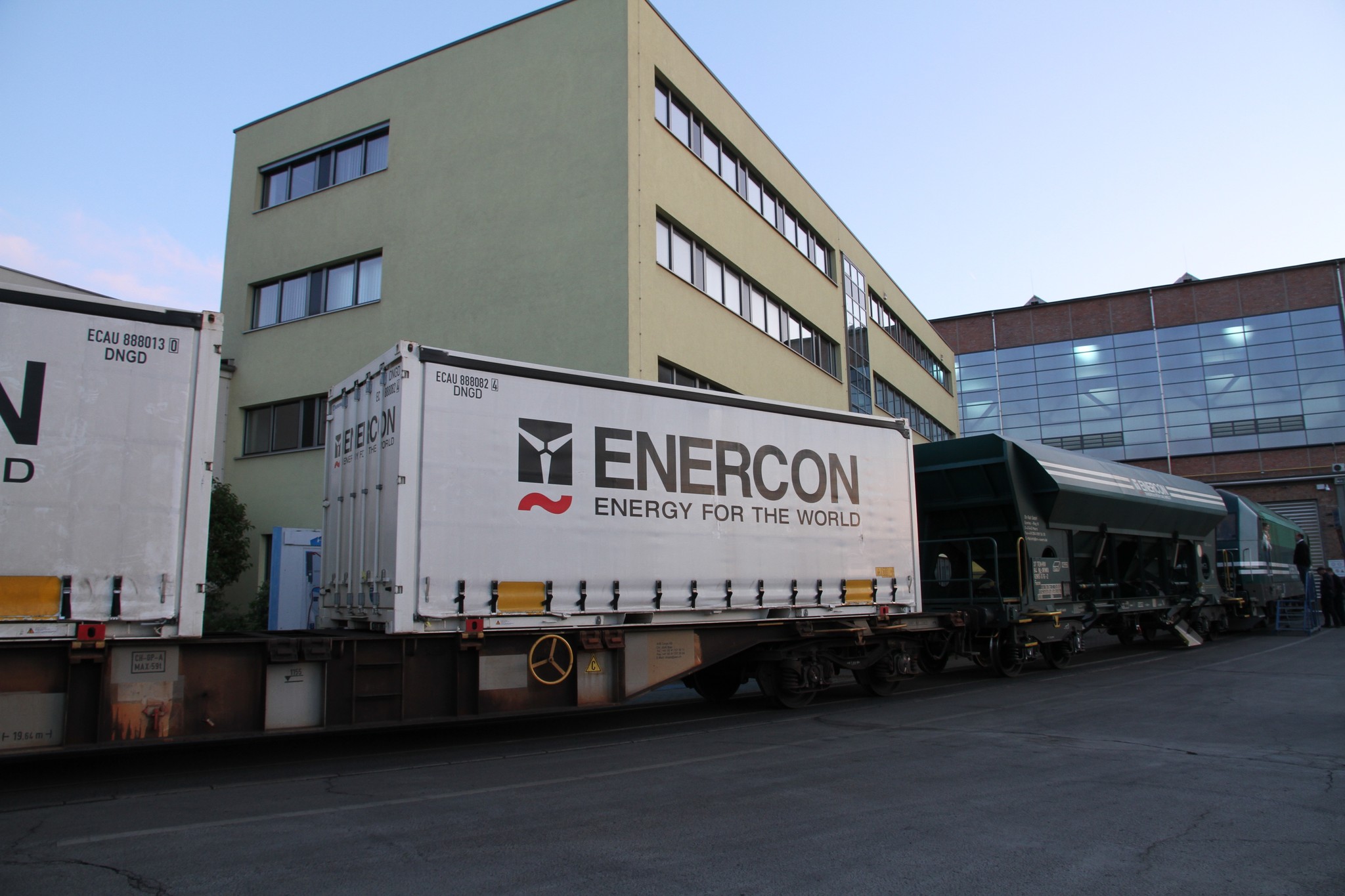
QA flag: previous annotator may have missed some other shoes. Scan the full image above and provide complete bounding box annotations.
[1331,625,1341,628]
[1321,625,1332,628]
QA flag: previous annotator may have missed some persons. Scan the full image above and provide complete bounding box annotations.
[1262,522,1272,551]
[1317,567,1345,628]
[1293,532,1311,588]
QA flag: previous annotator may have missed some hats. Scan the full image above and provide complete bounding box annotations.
[1315,567,1326,571]
